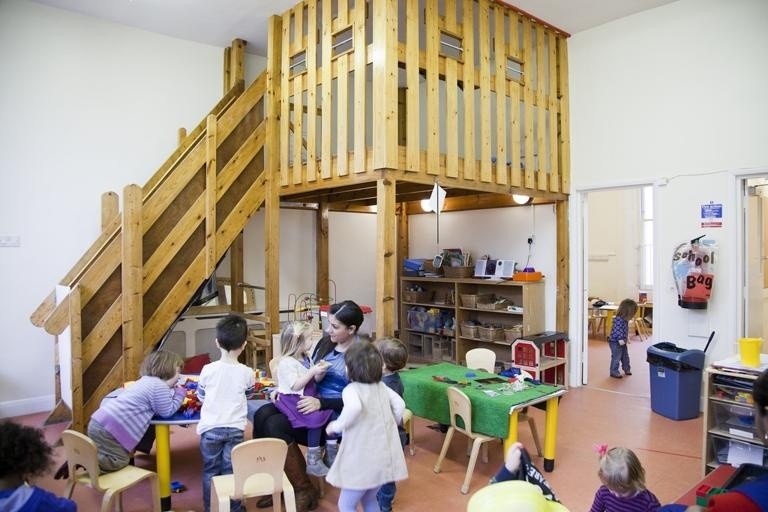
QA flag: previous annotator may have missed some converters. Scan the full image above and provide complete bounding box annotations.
[528,238,532,244]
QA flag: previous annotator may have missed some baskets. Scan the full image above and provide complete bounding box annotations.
[477,300,512,309]
[403,290,433,303]
[443,329,455,336]
[424,259,442,272]
[442,265,474,279]
[460,294,493,308]
[505,326,523,343]
[478,326,505,341]
[461,324,479,337]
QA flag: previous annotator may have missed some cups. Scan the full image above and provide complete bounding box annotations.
[738,338,764,368]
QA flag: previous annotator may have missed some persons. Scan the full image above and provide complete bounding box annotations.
[376,337,407,452]
[589,447,661,512]
[253,300,364,512]
[195,313,255,512]
[326,340,406,512]
[55,348,188,481]
[0,421,77,512]
[608,299,639,379]
[274,321,339,477]
[464,443,569,512]
[686,368,768,512]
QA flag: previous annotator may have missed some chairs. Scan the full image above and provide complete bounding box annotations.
[588,297,607,336]
[466,347,498,375]
[517,404,543,457]
[433,386,497,495]
[628,302,650,341]
[588,316,596,339]
[210,438,297,512]
[61,429,162,512]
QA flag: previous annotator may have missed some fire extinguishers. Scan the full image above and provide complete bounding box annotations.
[673,235,708,309]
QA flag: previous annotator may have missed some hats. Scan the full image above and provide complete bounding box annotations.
[466,481,570,512]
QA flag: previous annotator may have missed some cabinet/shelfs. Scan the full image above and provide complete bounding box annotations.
[510,330,569,386]
[399,276,545,369]
[700,354,768,480]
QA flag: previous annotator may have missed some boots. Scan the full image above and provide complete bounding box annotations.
[378,490,395,512]
[324,444,339,468]
[285,443,318,512]
[305,446,330,477]
[257,492,283,507]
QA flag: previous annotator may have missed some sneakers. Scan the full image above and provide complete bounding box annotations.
[622,367,632,375]
[610,373,622,378]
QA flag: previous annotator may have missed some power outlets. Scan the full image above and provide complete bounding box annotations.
[527,235,533,245]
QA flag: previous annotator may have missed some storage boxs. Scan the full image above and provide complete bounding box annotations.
[406,309,452,334]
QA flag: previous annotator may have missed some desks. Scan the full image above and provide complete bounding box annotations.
[400,361,568,473]
[123,375,275,511]
[588,301,653,341]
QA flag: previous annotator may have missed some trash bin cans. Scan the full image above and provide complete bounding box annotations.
[647,341,705,421]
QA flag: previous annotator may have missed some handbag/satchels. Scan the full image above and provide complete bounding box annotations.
[672,238,717,305]
[678,295,707,310]
